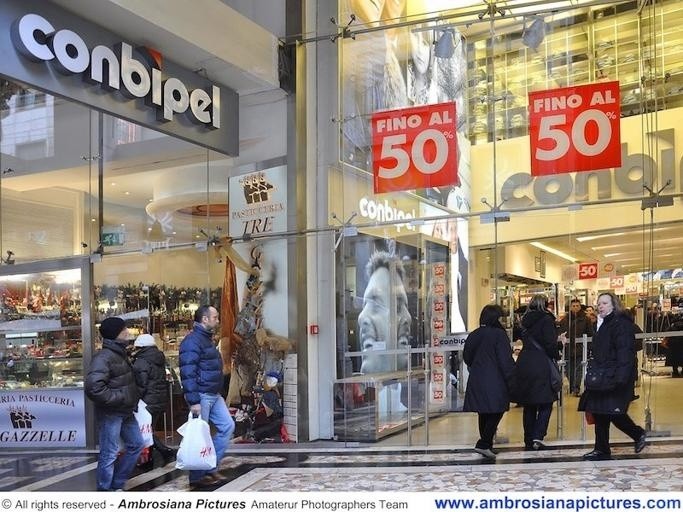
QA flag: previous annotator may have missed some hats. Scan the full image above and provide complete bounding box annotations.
[101,317,125,340]
[134,334,156,347]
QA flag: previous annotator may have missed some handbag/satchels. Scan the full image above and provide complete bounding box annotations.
[586,359,618,392]
[175,411,217,470]
[550,364,561,391]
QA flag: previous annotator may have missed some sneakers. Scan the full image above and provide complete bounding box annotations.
[475,448,495,458]
[525,439,547,448]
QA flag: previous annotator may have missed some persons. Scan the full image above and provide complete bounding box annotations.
[358,251,399,390]
[129,334,179,470]
[389,254,412,374]
[339,1,409,165]
[429,220,446,242]
[512,292,568,450]
[84,318,145,491]
[177,304,238,486]
[575,292,647,461]
[381,1,407,84]
[510,299,683,408]
[411,22,432,96]
[461,304,513,459]
[445,208,470,336]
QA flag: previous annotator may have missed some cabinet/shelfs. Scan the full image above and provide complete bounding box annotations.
[0,282,222,387]
[491,286,683,376]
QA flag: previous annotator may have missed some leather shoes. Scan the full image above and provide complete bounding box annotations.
[635,430,646,453]
[190,473,227,487]
[136,448,171,469]
[583,450,611,457]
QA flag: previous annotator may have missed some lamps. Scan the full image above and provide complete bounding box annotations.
[432,11,547,58]
